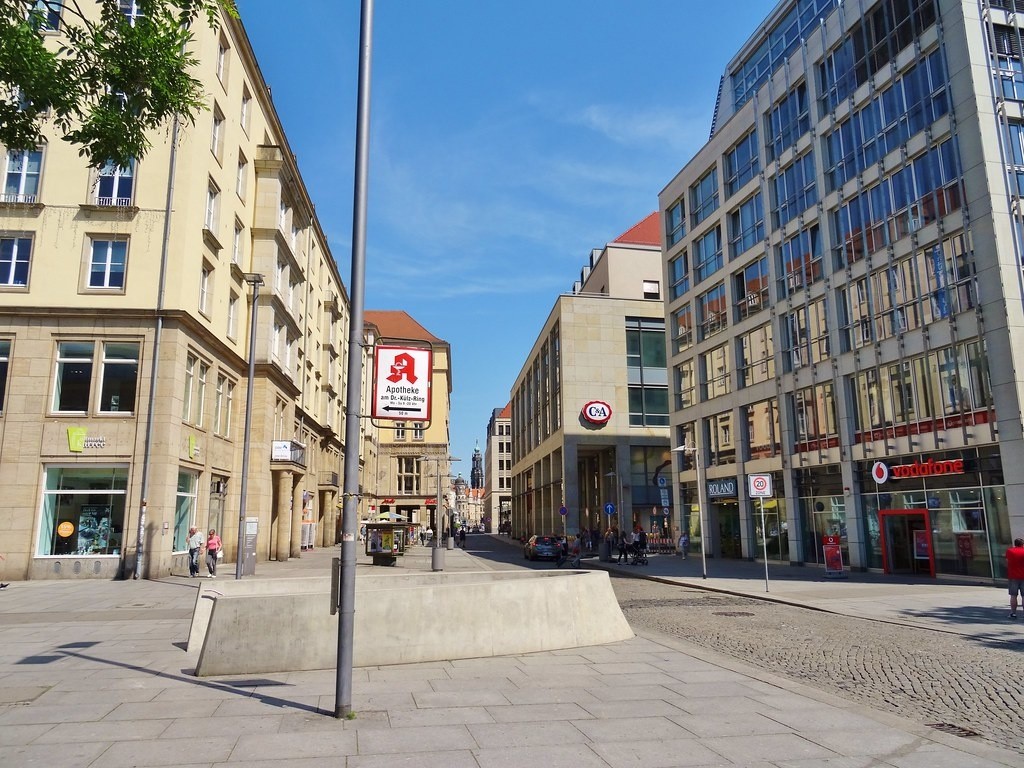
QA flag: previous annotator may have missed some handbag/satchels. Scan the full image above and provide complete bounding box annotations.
[217,550,223,558]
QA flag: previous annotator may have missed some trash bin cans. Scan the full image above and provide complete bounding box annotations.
[598,538,612,561]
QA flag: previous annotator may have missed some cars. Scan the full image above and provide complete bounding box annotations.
[481,524,486,530]
[426,529,433,540]
[472,526,479,533]
[524,535,562,561]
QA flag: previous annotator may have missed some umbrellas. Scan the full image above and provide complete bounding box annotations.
[374,512,408,522]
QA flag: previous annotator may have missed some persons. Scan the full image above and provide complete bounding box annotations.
[603,526,648,565]
[583,525,593,552]
[677,530,689,560]
[1004,538,1024,619]
[652,521,670,538]
[186,525,204,578]
[204,529,223,578]
[457,526,466,550]
[571,532,582,568]
[360,524,366,545]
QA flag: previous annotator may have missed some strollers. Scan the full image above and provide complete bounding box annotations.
[626,543,648,565]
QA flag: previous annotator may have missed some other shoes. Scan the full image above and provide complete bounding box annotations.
[617,564,622,566]
[207,574,212,577]
[626,564,630,566]
[212,576,216,578]
[1008,612,1017,619]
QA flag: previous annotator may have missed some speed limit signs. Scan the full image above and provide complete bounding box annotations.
[748,473,773,498]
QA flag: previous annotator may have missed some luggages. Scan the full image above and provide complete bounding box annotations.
[555,553,574,568]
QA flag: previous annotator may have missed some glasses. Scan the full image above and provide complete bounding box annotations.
[209,533,214,535]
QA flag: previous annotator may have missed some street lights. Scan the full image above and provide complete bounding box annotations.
[669,445,707,579]
[236,272,266,579]
[416,455,461,571]
